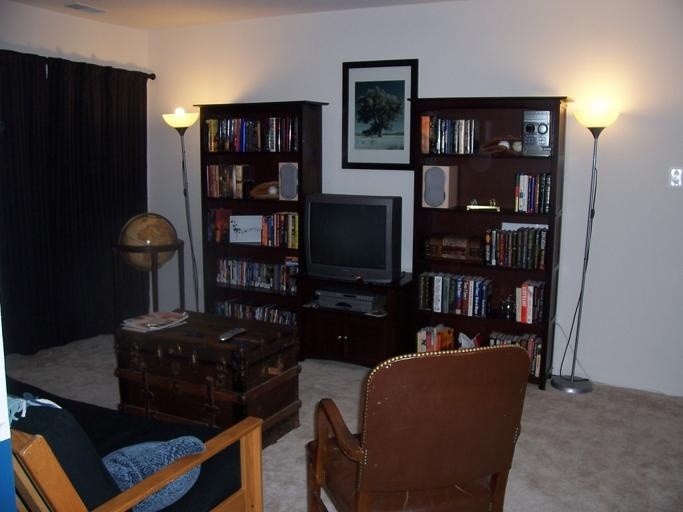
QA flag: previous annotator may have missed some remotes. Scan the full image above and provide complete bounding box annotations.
[218,327,245,341]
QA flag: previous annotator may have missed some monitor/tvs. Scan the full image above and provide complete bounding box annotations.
[305,193,402,284]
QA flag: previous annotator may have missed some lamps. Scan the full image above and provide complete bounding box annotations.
[161,106,200,314]
[551,94,620,394]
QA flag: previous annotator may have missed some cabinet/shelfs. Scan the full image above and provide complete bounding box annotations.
[195,101,329,330]
[289,272,413,367]
[405,97,575,391]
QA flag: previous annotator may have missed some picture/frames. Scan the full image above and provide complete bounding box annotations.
[341,58,419,170]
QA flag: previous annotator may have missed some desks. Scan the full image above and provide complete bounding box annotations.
[114,309,301,455]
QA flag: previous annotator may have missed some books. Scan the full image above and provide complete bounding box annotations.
[416,114,551,379]
[122,309,191,333]
[206,116,298,326]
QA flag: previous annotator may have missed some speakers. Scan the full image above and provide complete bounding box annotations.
[422,166,460,209]
[278,162,298,201]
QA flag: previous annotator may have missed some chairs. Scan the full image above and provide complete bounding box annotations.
[304,345,530,512]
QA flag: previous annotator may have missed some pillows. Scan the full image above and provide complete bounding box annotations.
[101,435,206,512]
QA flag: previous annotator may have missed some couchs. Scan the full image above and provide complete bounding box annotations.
[6,374,263,512]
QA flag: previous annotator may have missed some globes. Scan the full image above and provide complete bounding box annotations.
[110,212,185,330]
